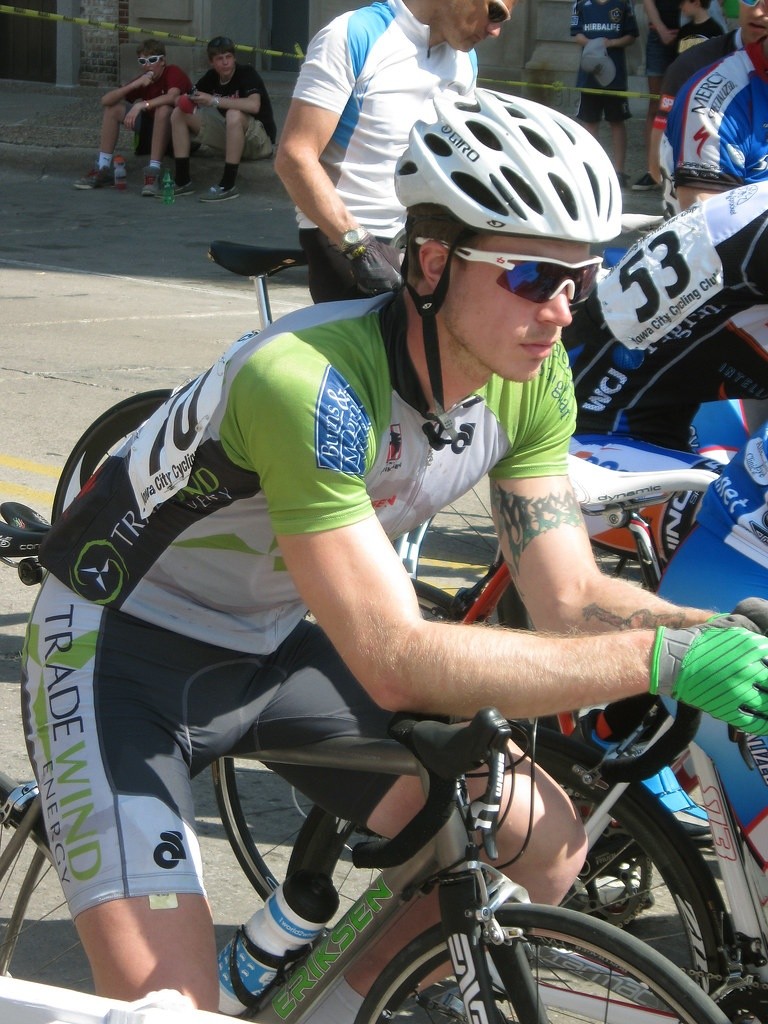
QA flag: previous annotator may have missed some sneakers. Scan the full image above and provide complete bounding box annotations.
[198,185,240,202]
[141,165,161,196]
[71,158,115,190]
[155,182,192,199]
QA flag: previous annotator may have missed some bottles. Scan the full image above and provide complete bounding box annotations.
[162,168,175,205]
[213,867,340,1016]
[114,162,128,189]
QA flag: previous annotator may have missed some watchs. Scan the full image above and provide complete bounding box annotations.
[144,101,151,109]
[339,226,368,253]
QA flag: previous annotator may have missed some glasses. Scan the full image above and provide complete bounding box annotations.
[137,54,164,66]
[487,1,513,26]
[740,0,762,7]
[412,237,602,304]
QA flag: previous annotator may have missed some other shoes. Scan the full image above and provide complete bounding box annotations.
[632,173,662,190]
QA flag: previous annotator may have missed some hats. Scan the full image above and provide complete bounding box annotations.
[581,36,616,87]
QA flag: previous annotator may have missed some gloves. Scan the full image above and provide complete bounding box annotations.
[648,611,768,738]
[342,233,405,296]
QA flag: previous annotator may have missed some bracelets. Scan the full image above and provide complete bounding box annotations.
[213,97,220,107]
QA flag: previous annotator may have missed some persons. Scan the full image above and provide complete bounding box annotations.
[275,0,513,304]
[73,36,277,203]
[653,424,768,912]
[559,0,768,453]
[21,90,768,1024]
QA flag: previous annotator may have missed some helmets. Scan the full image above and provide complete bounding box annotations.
[393,86,621,244]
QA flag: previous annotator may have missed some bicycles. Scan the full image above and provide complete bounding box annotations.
[0,708,731,1024]
[48,244,730,1014]
